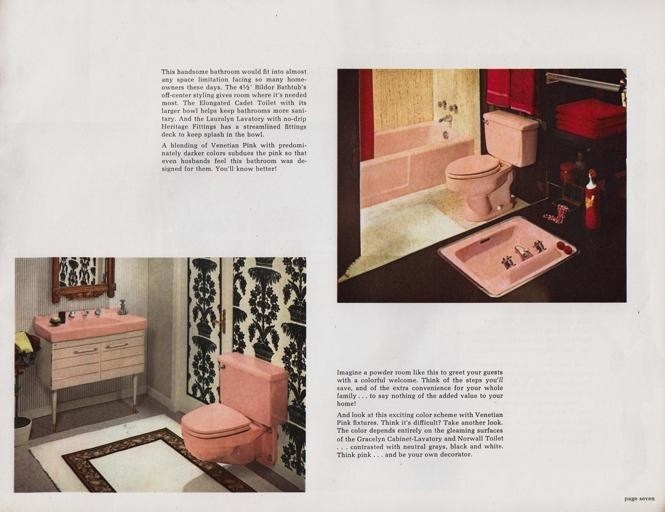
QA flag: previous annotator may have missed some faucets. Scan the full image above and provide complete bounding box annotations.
[118,299,128,314]
[83,310,90,316]
[514,244,534,261]
[439,112,453,123]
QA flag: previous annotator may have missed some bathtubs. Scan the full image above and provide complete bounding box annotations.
[360,119,474,209]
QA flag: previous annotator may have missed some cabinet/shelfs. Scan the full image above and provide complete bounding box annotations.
[34,329,145,435]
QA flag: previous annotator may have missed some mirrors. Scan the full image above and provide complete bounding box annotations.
[53,258,116,304]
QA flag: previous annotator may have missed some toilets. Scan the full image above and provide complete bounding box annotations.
[181,353,290,468]
[445,107,539,222]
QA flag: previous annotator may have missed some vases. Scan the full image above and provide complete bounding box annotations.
[15,415,32,447]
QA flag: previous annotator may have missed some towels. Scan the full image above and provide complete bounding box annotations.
[16,331,34,354]
[483,70,537,114]
[554,99,627,140]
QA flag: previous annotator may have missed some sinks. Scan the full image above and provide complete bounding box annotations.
[69,316,121,329]
[437,212,578,298]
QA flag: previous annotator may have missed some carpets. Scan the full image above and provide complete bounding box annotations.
[30,414,286,492]
